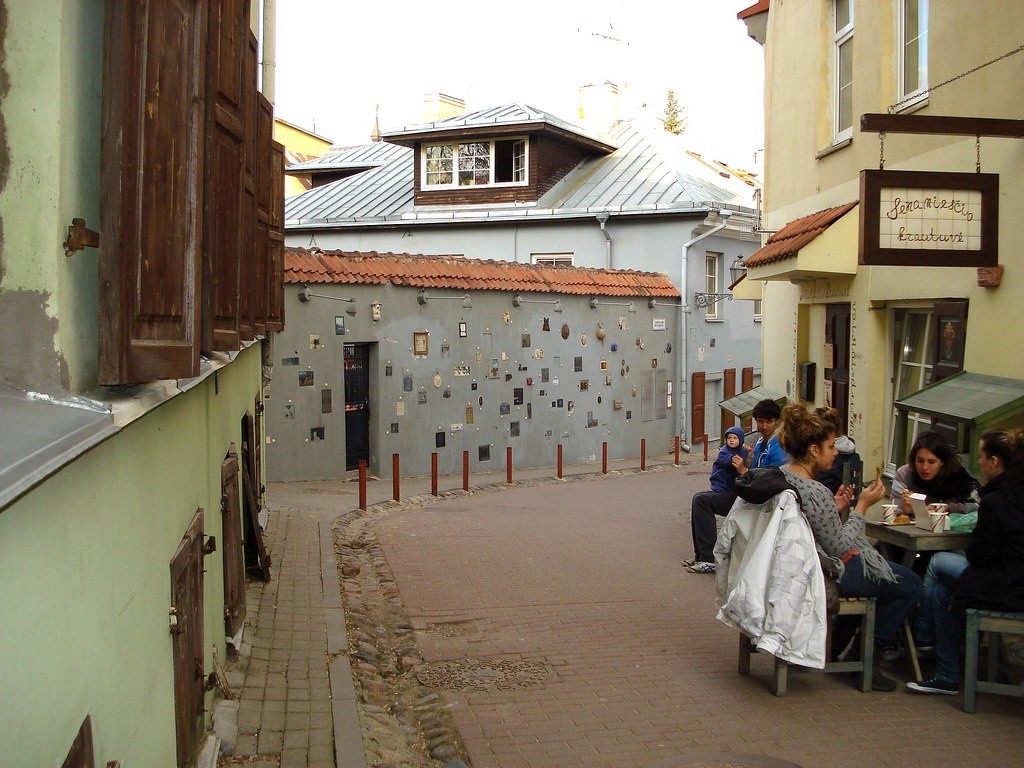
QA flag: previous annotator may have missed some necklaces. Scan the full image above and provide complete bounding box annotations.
[794,461,812,478]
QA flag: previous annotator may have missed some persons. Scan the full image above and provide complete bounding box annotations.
[709,427,753,494]
[812,406,855,497]
[777,405,980,692]
[899,431,1024,694]
[682,399,790,573]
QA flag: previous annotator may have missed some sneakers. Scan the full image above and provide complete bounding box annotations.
[906,676,960,695]
[915,640,935,651]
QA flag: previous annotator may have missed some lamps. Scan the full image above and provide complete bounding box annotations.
[296,284,356,318]
[589,298,636,313]
[694,253,747,311]
[648,298,691,313]
[417,291,472,309]
[512,295,562,314]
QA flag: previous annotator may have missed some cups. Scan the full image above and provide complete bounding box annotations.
[931,503,948,513]
[882,505,898,522]
[929,513,944,534]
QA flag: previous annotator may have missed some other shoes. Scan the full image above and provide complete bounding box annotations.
[882,645,896,660]
[685,561,716,573]
[681,557,695,566]
[851,656,896,691]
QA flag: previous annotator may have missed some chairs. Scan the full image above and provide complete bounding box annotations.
[738,489,876,698]
[841,453,863,524]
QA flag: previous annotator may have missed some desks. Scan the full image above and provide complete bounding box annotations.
[865,520,977,685]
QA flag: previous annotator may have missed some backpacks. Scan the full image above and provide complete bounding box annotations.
[819,555,840,614]
